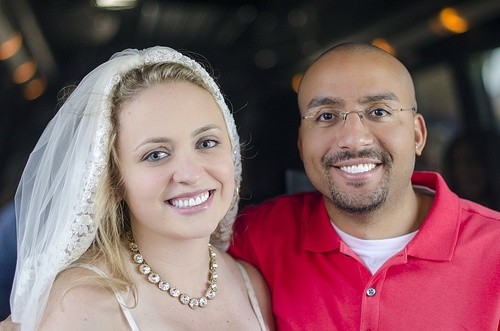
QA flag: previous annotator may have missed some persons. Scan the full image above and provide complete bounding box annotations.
[10,45,277,331]
[0,42,500,331]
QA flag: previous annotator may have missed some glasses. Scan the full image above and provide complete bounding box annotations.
[301,103,417,125]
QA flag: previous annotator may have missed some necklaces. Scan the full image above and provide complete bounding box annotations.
[121,219,219,310]
